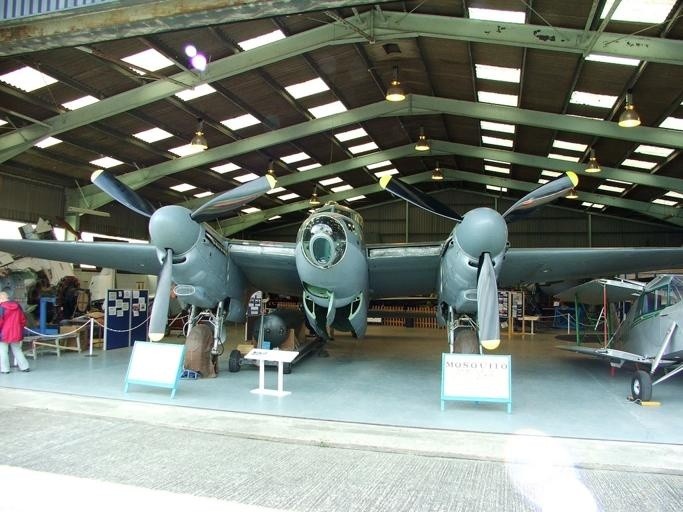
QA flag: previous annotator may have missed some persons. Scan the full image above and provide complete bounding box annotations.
[0,290,33,373]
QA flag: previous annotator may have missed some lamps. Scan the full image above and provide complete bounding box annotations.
[188,117,323,206]
[585,87,642,174]
[385,61,444,180]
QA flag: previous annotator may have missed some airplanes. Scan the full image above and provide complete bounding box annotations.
[550,270,683,407]
[0,165,683,376]
